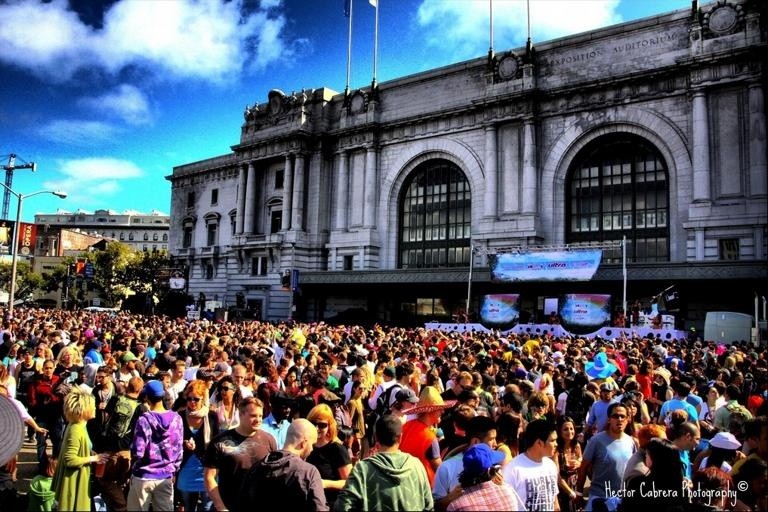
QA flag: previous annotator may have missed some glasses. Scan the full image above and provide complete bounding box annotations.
[219,386,233,391]
[186,397,204,402]
[310,422,328,429]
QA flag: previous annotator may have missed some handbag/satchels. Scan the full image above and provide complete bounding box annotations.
[377,385,402,416]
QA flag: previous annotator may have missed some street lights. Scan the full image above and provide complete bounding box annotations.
[0,182,69,324]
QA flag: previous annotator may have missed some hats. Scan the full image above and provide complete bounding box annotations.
[600,383,614,391]
[463,444,504,472]
[269,392,295,403]
[395,389,419,403]
[750,352,758,360]
[318,392,343,401]
[145,380,163,397]
[747,396,764,408]
[429,346,438,353]
[214,363,227,376]
[0,393,25,467]
[144,347,156,360]
[584,352,618,378]
[404,386,458,415]
[197,369,215,379]
[383,366,396,378]
[708,432,741,449]
[91,341,104,348]
[654,368,671,387]
[120,351,139,361]
[24,348,33,355]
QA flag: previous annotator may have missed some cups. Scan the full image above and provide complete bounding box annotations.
[565,453,577,473]
[175,505,185,512]
[95,460,106,477]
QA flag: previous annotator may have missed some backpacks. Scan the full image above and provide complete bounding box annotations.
[725,405,749,440]
[104,396,141,437]
